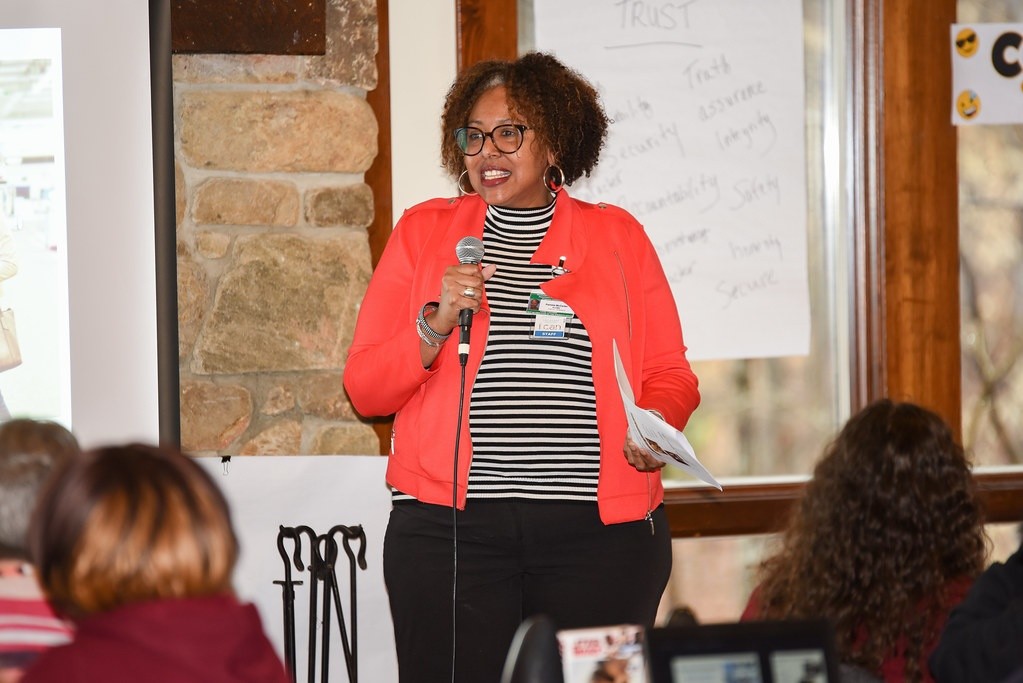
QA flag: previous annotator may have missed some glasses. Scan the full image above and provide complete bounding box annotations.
[454,124,535,156]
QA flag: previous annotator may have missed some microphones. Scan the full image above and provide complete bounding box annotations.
[455,237,485,366]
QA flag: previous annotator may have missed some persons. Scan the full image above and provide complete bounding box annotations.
[737,396,992,683]
[1,416,288,682]
[926,542,1023,683]
[342,51,702,683]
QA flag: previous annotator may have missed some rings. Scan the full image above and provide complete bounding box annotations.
[463,286,475,299]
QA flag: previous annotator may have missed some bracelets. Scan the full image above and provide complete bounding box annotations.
[415,301,453,347]
[645,409,666,422]
